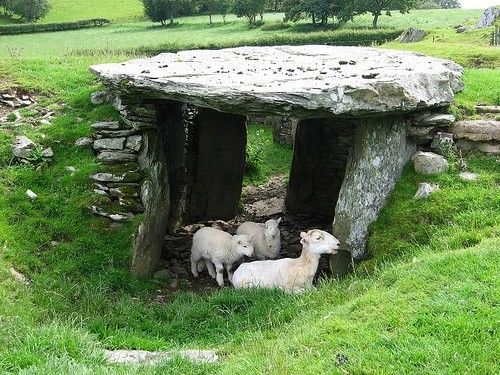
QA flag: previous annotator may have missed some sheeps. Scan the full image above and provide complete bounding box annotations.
[231,228,341,295]
[189,226,255,287]
[235,216,283,262]
